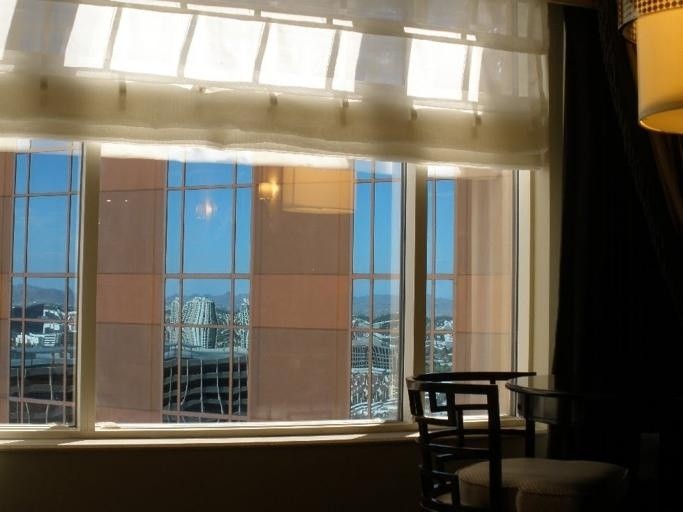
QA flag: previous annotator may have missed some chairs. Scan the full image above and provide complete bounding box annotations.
[404,367,622,511]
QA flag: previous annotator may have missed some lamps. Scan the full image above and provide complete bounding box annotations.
[282,165,356,216]
[612,1,682,139]
[257,181,276,202]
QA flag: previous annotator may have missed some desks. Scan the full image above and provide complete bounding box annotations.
[503,374,568,458]
